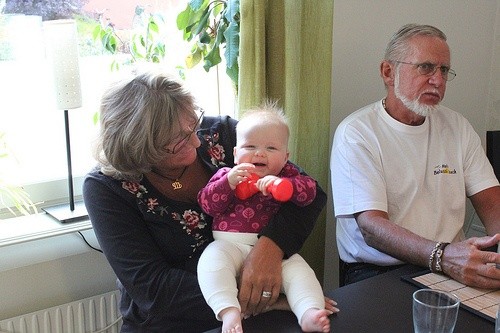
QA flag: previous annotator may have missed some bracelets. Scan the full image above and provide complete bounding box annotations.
[436,243,453,274]
[429,242,444,272]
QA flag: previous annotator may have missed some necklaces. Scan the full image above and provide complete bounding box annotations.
[383,96,424,125]
[152,167,188,190]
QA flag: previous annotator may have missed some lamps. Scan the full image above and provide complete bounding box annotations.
[43,19,99,223]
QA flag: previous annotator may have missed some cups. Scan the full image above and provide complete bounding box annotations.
[413,289,460,333]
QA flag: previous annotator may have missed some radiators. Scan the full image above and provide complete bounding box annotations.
[0,289,123,333]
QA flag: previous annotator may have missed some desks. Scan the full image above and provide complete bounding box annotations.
[207,265,500,333]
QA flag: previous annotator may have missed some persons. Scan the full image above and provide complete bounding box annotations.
[196,111,331,333]
[82,73,339,333]
[330,23,500,289]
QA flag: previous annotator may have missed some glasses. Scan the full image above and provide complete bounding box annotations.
[159,106,205,154]
[388,59,456,82]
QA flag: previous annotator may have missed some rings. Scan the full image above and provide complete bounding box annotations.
[262,291,272,297]
